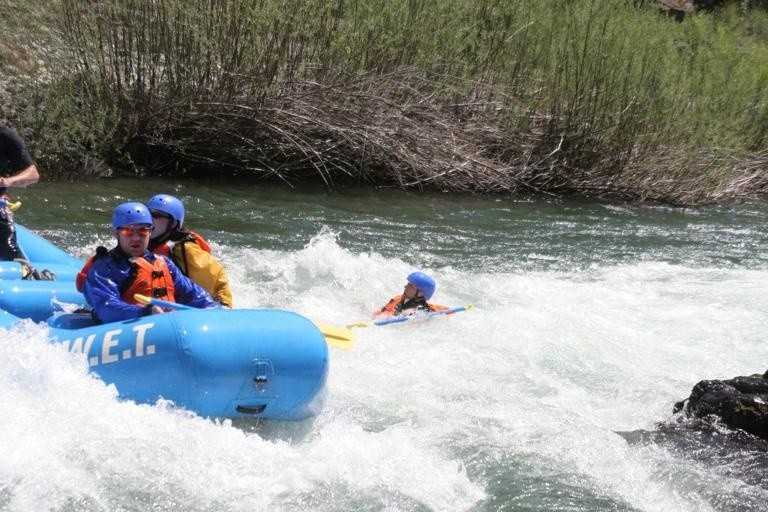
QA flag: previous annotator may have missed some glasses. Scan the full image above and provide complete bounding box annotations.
[149,211,172,221]
[116,226,151,237]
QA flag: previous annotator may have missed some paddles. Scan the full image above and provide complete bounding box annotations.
[134,294,356,349]
[346,304,473,328]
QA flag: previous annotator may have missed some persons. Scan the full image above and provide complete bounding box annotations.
[147,194,235,309]
[74,203,220,320]
[0,122,41,262]
[369,271,443,326]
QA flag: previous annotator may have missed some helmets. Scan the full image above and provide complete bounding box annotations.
[406,271,437,300]
[112,201,153,230]
[145,192,185,229]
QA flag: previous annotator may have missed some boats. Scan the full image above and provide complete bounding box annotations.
[0,221,331,420]
